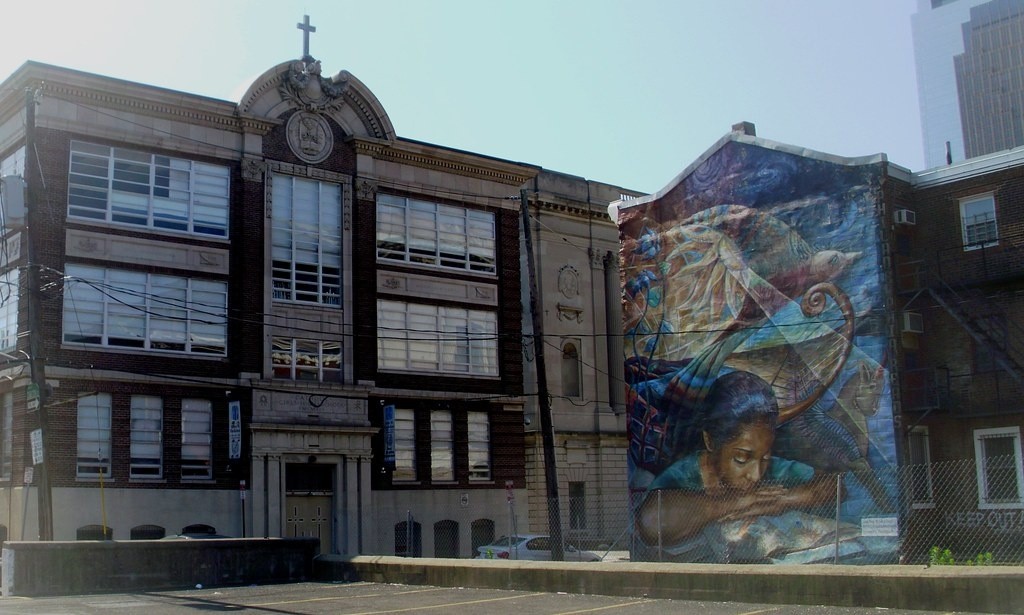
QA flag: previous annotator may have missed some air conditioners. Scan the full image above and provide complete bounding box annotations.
[897,312,924,333]
[894,209,916,226]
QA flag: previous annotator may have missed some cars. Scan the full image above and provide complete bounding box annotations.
[474,533,601,561]
